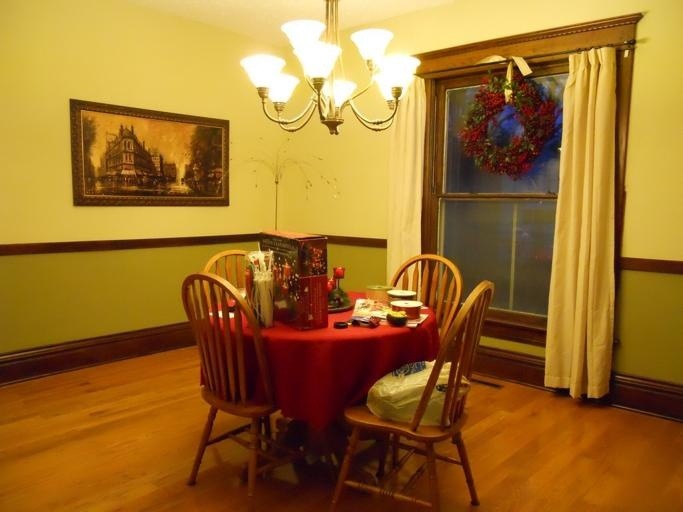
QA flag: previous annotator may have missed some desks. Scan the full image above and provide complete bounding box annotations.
[202,288,439,472]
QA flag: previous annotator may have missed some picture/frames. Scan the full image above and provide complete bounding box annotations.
[69,99,229,207]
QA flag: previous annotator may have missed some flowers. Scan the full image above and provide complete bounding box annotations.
[456,66,563,182]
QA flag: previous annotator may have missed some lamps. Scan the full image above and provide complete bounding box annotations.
[236,2,422,136]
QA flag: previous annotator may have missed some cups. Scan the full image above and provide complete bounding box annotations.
[254,272,274,327]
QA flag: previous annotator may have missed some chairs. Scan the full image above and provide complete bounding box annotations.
[388,254,463,346]
[204,248,254,294]
[180,271,281,497]
[331,280,494,512]
